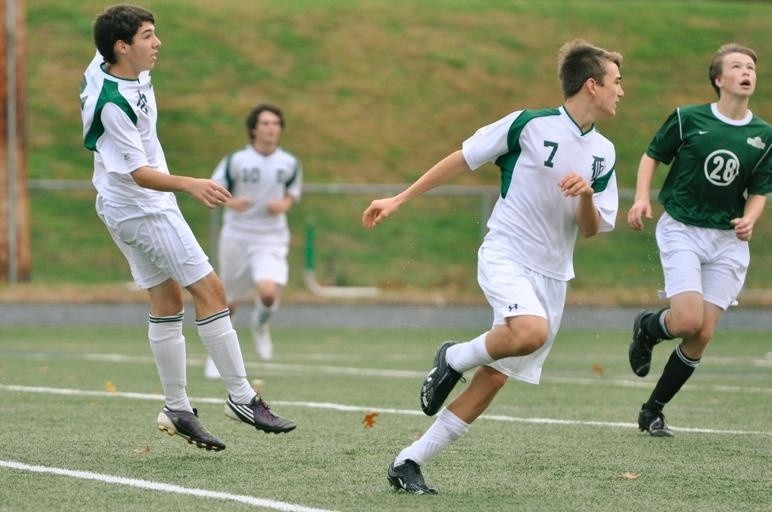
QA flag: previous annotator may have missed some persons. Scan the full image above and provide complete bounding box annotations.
[203,103,304,379]
[362,38,624,495]
[627,43,772,437]
[79,4,298,452]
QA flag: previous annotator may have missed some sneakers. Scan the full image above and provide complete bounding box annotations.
[204,357,220,379]
[420,341,466,416]
[387,459,438,495]
[638,403,674,437]
[224,392,297,435]
[156,406,225,452]
[629,309,658,377]
[251,322,272,361]
